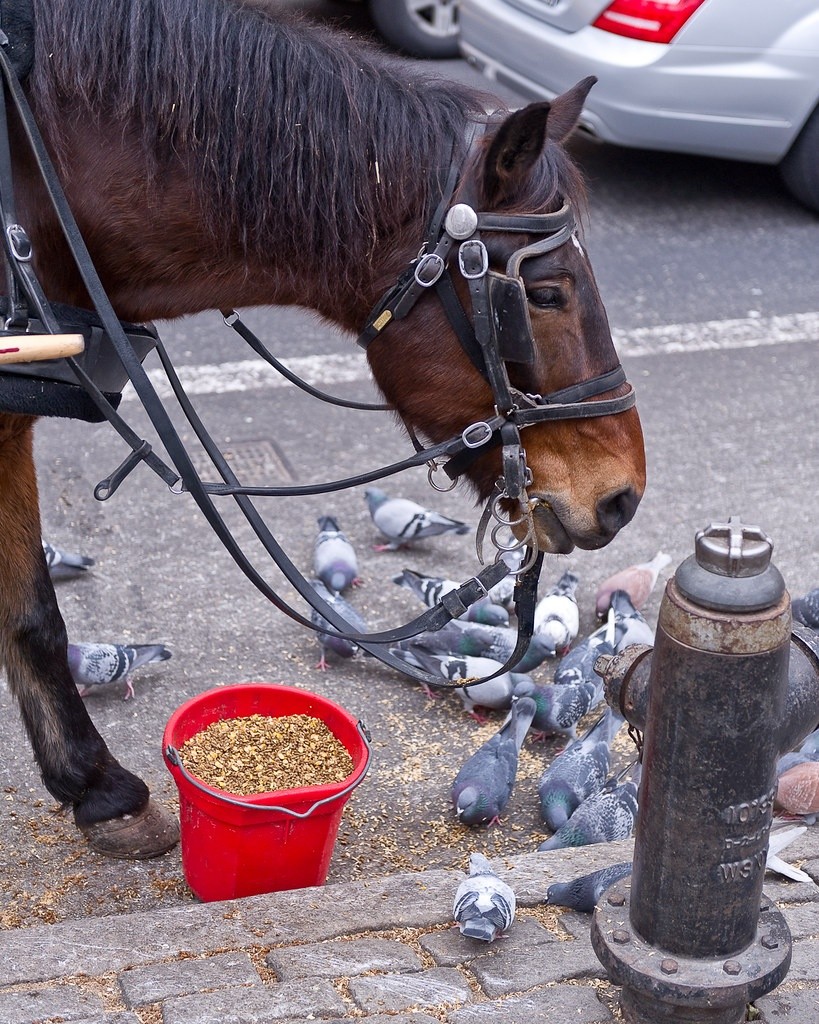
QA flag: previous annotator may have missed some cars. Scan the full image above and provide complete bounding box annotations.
[298,0,819,217]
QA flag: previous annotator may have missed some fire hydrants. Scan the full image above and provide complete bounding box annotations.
[589,516,819,1023]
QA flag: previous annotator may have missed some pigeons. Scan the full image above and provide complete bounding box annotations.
[529,570,580,656]
[41,539,95,570]
[363,487,470,553]
[66,643,172,700]
[309,577,372,672]
[451,695,538,831]
[388,645,534,729]
[531,761,643,854]
[596,551,673,621]
[536,707,625,834]
[765,728,819,884]
[553,590,656,708]
[540,862,634,912]
[396,617,556,674]
[512,679,596,757]
[392,568,509,630]
[488,535,529,614]
[453,851,517,942]
[313,515,365,599]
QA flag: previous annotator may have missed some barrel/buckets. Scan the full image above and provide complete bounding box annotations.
[162,683,374,903]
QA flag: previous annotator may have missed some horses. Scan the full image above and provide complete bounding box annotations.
[0,0,648,861]
